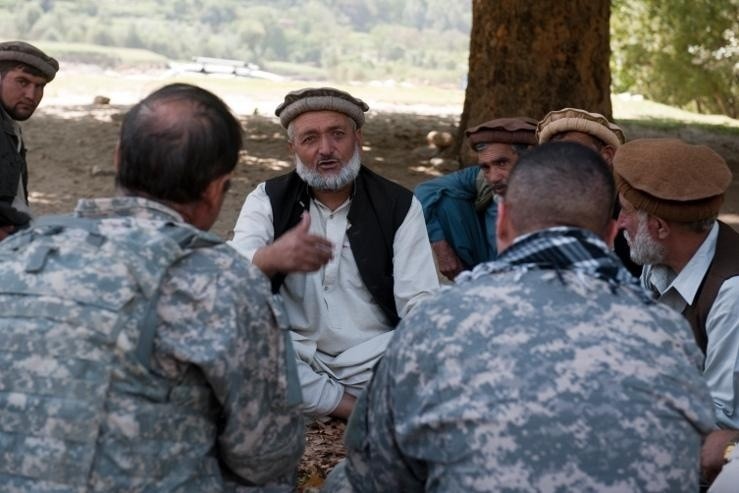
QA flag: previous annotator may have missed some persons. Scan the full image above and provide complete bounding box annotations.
[227,87,440,421]
[0,39,60,246]
[534,107,644,281]
[315,138,716,492]
[410,116,538,282]
[0,78,307,492]
[610,135,739,492]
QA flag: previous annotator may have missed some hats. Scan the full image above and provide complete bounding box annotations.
[0,39,60,84]
[533,106,625,153]
[273,85,372,131]
[466,114,539,148]
[612,137,734,223]
